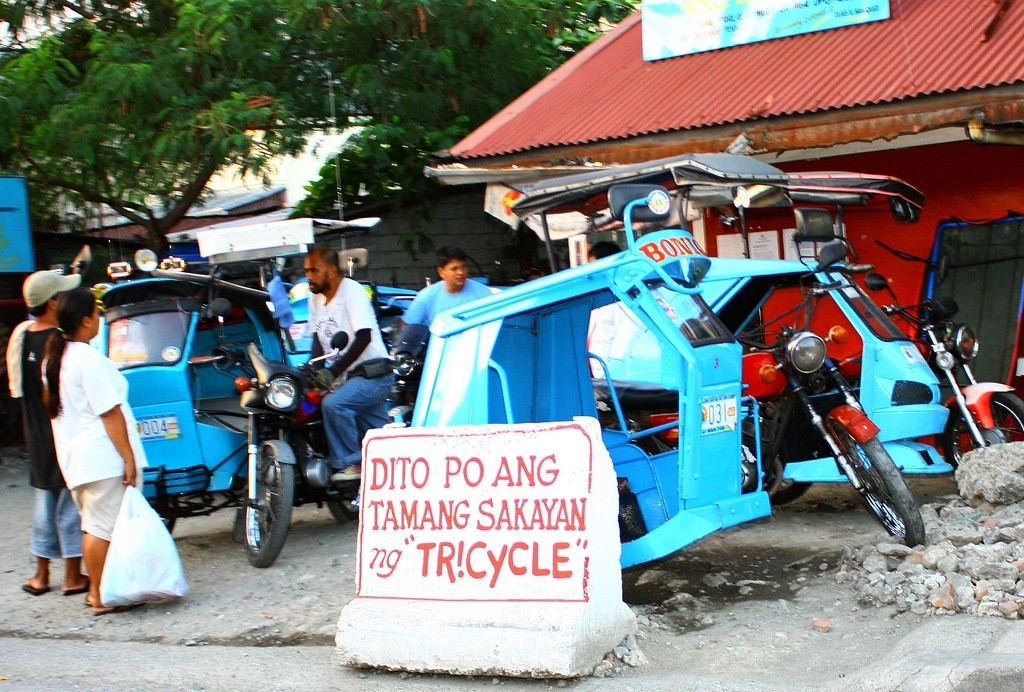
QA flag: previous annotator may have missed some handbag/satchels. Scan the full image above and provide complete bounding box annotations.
[347,357,393,379]
[99,486,189,605]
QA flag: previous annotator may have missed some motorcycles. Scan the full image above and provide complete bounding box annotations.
[87,151,1024,569]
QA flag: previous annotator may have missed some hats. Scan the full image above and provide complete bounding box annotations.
[23,270,81,308]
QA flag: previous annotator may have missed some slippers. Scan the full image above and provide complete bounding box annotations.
[64,576,89,595]
[21,579,51,595]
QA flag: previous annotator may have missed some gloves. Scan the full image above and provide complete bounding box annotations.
[312,368,334,391]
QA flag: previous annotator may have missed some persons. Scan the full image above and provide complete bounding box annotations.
[303,247,394,509]
[587,241,621,263]
[42,286,147,616]
[8,270,91,596]
[390,245,492,358]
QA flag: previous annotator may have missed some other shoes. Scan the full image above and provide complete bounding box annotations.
[92,607,114,615]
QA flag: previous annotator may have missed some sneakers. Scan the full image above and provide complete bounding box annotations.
[330,463,361,480]
